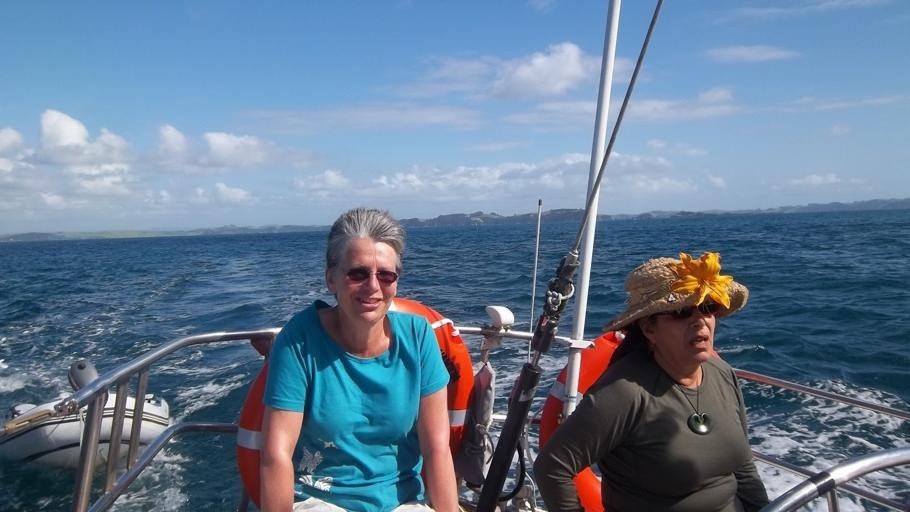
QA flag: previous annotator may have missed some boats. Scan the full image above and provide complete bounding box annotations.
[0,359,170,474]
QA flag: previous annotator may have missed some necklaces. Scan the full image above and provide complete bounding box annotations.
[680,378,714,435]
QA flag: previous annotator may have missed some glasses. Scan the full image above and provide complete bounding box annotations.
[653,305,717,319]
[346,268,397,284]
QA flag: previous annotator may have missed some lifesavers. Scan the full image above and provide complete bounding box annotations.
[538,329,722,512]
[237,297,475,512]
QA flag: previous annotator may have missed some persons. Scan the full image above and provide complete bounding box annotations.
[259,209,458,512]
[533,252,769,512]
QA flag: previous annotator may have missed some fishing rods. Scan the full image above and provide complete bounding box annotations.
[476,0,662,512]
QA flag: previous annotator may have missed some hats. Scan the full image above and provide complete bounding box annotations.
[601,256,749,332]
